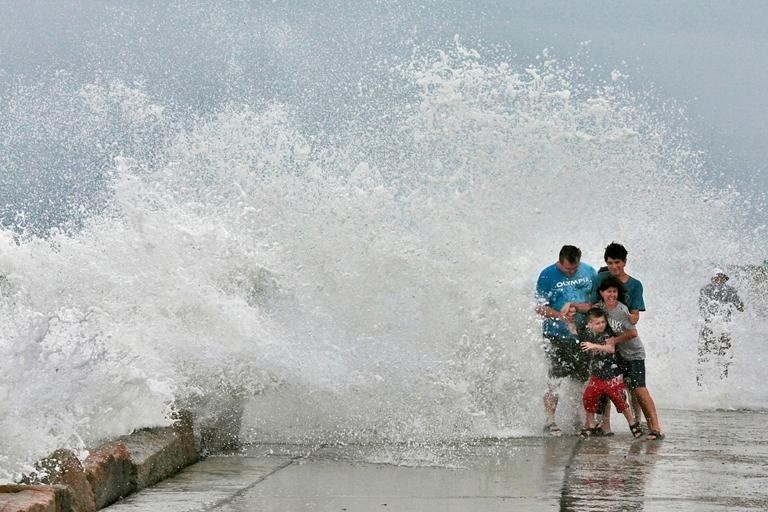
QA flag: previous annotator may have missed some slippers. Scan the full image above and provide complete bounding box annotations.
[646,431,664,440]
[544,423,561,436]
[578,427,614,441]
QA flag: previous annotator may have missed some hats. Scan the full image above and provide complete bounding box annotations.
[711,268,729,282]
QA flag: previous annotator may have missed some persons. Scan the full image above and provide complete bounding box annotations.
[533,240,666,441]
[696,267,744,391]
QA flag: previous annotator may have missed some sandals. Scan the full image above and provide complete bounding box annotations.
[630,423,642,437]
